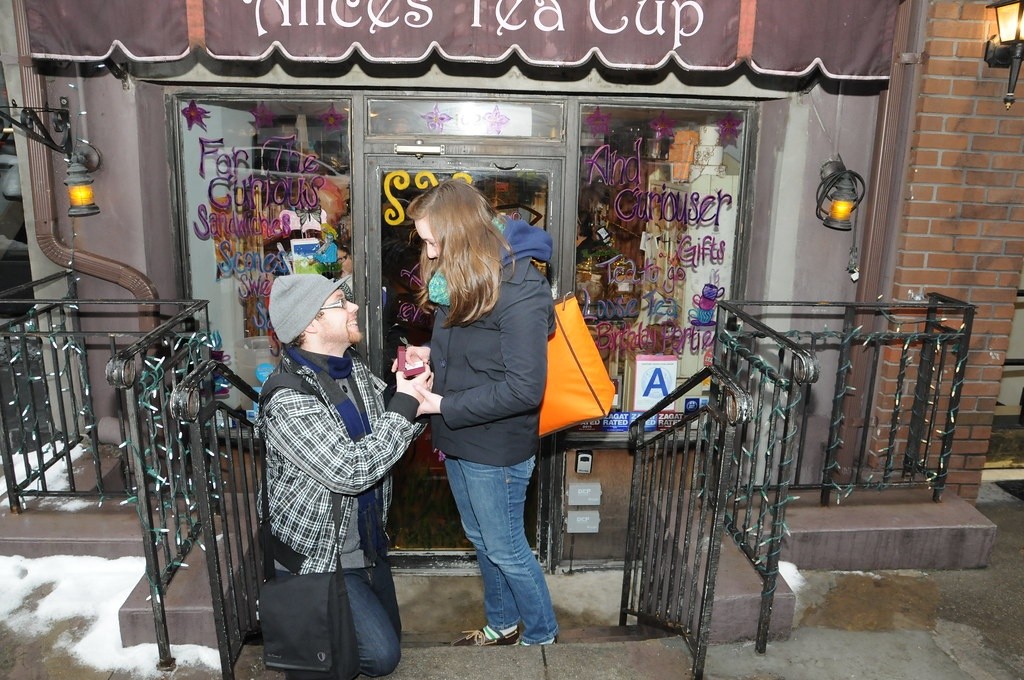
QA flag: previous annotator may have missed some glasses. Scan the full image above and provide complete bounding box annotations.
[320,298,348,311]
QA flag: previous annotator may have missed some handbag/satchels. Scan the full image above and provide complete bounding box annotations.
[532,292,615,437]
[260,570,360,680]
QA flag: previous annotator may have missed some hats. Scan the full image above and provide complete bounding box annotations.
[268,272,350,344]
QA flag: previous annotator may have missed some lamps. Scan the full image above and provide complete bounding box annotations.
[819,161,858,230]
[984,0,1024,110]
[64,139,102,217]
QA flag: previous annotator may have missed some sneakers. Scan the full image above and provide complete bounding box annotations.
[451,625,521,649]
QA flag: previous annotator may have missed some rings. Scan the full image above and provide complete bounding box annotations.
[413,364,415,367]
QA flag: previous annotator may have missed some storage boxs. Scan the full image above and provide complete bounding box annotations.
[398,345,425,378]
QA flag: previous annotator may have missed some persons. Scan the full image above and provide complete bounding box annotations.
[256,274,434,677]
[391,179,559,647]
[576,218,590,264]
